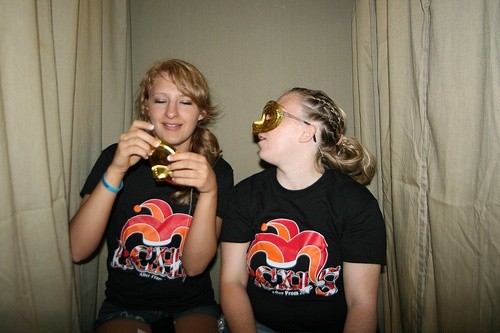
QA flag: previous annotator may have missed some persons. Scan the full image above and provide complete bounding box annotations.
[219,88,386,333]
[68,58,234,333]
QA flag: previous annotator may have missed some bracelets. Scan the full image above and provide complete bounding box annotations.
[101,172,123,192]
